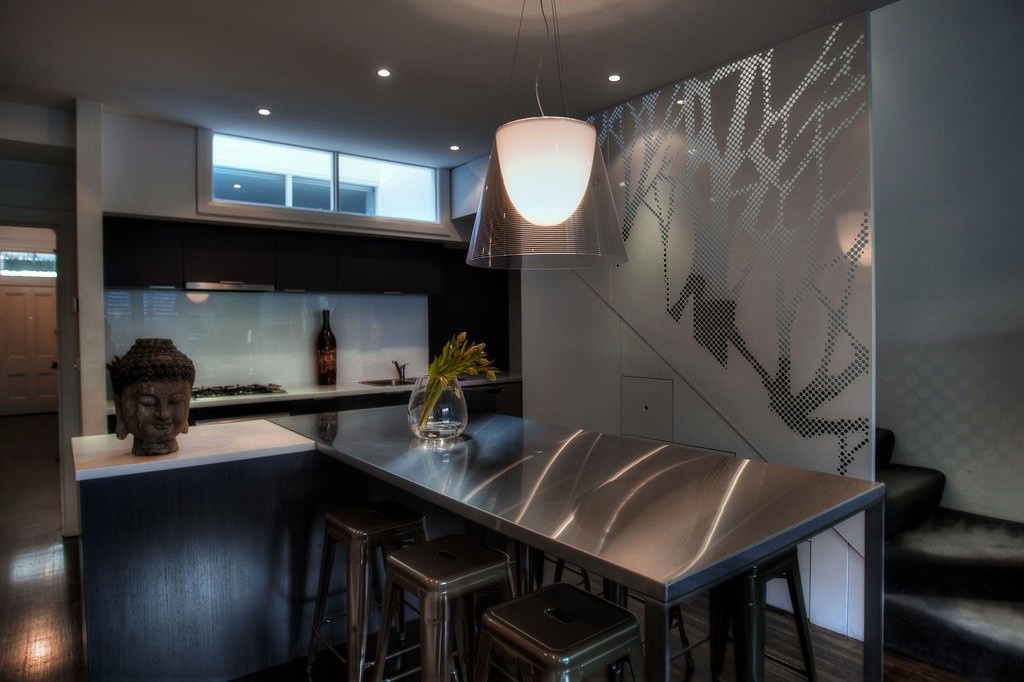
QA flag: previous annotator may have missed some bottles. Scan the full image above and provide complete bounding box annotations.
[317,310,337,385]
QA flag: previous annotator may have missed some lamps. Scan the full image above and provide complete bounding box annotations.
[465,0,633,269]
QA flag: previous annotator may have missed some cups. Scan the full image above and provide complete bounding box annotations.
[407,375,469,440]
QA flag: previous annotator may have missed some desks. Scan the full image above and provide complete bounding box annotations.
[68,418,318,481]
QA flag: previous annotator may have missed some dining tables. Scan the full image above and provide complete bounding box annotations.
[265,402,888,681]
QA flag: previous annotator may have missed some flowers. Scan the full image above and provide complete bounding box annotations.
[417,331,502,431]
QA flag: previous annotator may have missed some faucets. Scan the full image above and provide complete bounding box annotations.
[391,358,412,383]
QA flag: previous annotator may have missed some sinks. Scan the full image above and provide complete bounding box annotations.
[359,378,420,388]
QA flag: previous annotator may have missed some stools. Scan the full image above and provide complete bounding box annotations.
[299,500,828,682]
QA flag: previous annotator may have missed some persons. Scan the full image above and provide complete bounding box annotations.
[109,337,195,456]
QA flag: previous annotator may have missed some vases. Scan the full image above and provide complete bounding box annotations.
[407,373,470,440]
